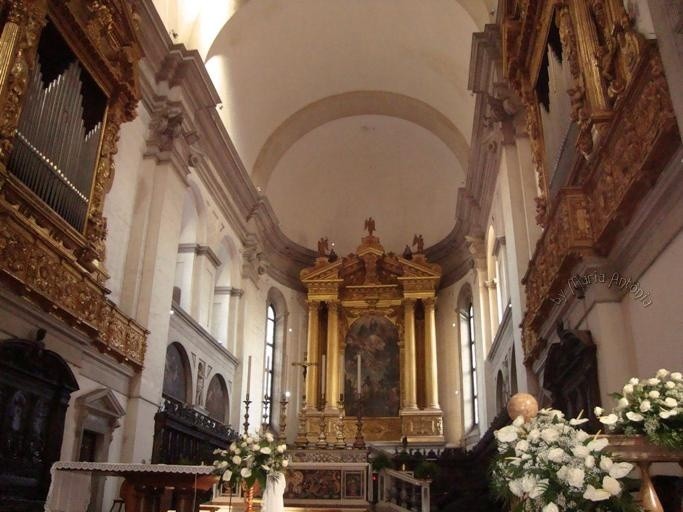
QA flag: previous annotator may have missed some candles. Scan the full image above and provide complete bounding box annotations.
[244,352,270,395]
[320,351,361,397]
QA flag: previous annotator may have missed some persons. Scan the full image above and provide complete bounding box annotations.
[316,215,425,417]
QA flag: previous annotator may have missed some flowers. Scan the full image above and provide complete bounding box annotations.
[481,364,683,511]
[207,424,289,489]
